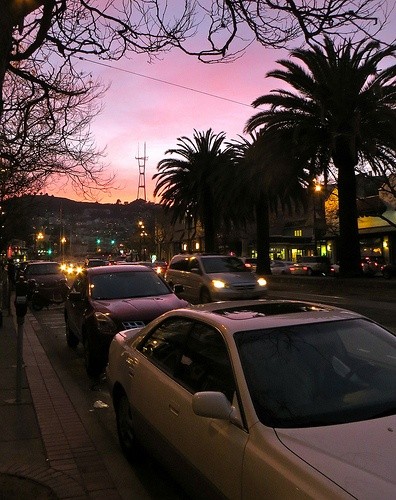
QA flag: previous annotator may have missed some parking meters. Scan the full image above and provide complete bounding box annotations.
[13,283,28,404]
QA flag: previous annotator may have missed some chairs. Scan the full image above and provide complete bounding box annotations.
[246,366,291,412]
[311,341,365,400]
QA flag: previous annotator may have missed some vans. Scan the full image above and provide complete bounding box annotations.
[163,252,270,304]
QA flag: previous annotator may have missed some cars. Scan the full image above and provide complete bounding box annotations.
[242,253,396,281]
[62,263,197,367]
[12,255,169,311]
[103,298,396,500]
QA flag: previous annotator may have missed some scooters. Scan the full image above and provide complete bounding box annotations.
[30,279,70,312]
